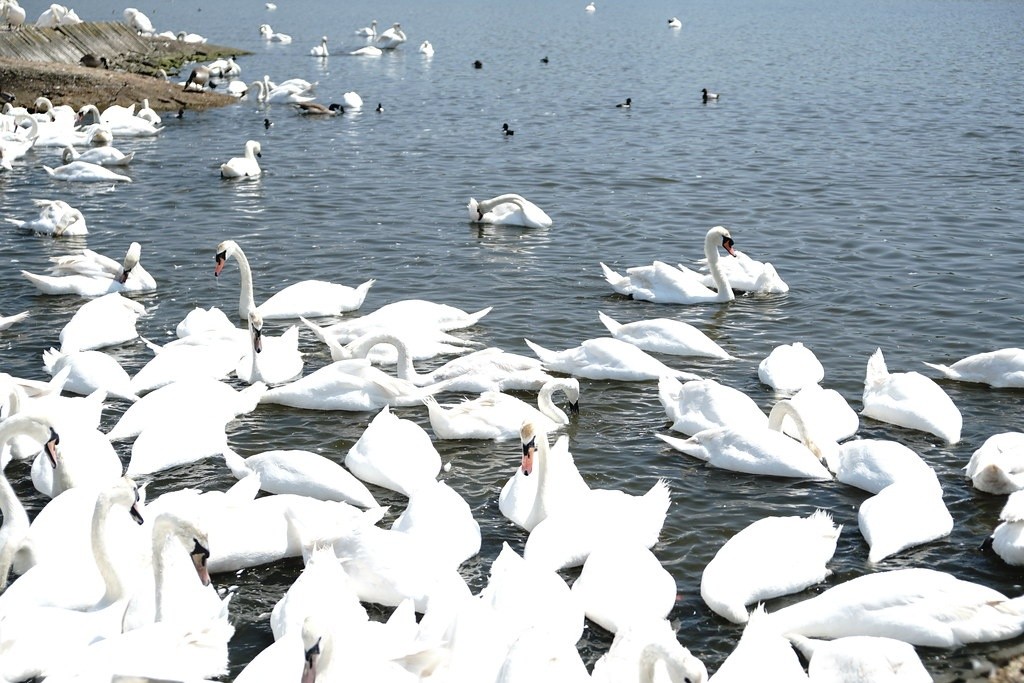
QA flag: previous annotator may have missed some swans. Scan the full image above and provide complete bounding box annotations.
[13,113,94,147]
[19,241,157,300]
[0,362,108,430]
[62,146,136,165]
[219,140,262,181]
[0,0,435,128]
[465,192,552,228]
[81,123,113,142]
[259,299,1024,447]
[3,198,89,237]
[0,291,304,480]
[33,96,77,123]
[78,103,166,136]
[43,161,132,183]
[0,102,52,171]
[0,415,1024,683]
[136,98,161,124]
[215,239,375,320]
[599,226,788,304]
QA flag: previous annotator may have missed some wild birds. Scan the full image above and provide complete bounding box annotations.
[470,2,720,136]
